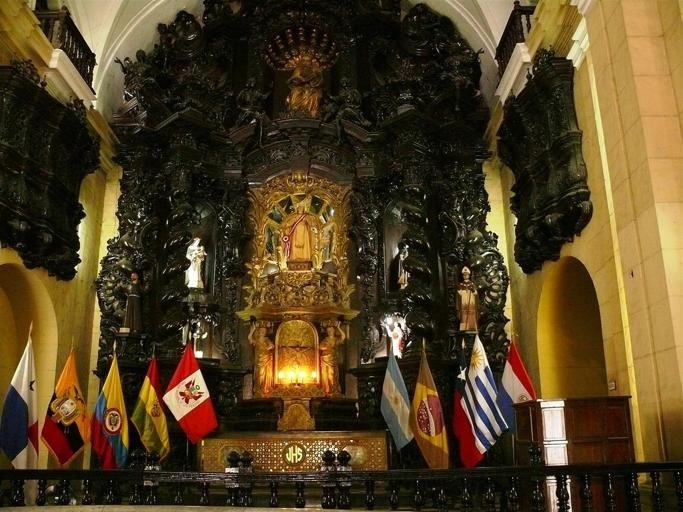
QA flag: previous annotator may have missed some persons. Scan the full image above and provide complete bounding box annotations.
[245,322,276,398]
[186,319,208,355]
[453,265,479,333]
[284,51,322,118]
[182,237,209,291]
[317,320,346,396]
[394,244,414,291]
[233,75,274,126]
[324,73,367,127]
[275,201,326,264]
[117,269,153,335]
[385,320,403,358]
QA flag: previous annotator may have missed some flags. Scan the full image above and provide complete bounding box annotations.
[449,334,517,470]
[0,336,40,506]
[129,356,168,468]
[378,349,415,453]
[160,341,221,446]
[405,344,452,470]
[41,336,94,472]
[499,343,538,406]
[88,353,130,476]
